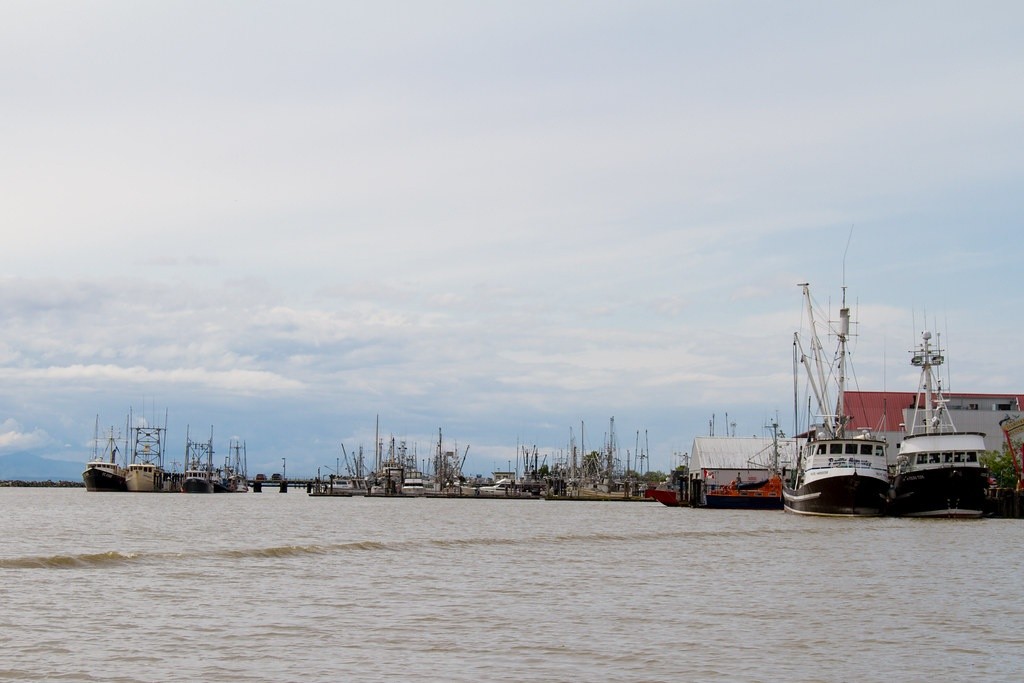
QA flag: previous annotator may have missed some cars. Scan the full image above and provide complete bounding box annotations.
[272,474,282,480]
[256,474,267,480]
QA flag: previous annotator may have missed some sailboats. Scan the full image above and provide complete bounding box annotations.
[886,304,989,520]
[782,282,891,518]
[82,412,127,492]
[123,394,168,492]
[182,424,217,493]
[307,413,650,501]
[705,423,785,511]
[161,459,183,493]
[213,439,250,494]
[646,452,690,507]
